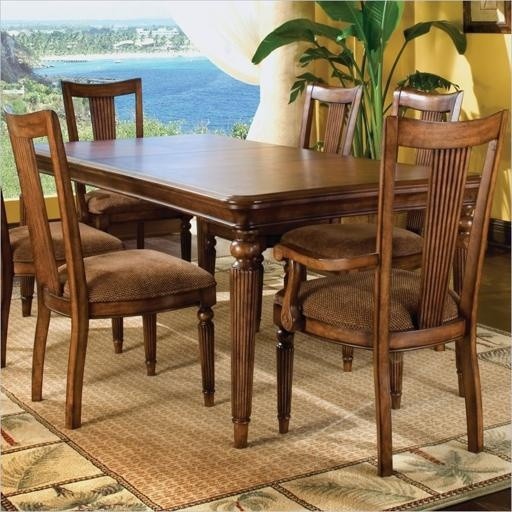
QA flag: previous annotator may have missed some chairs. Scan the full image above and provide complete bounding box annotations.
[273,108,510,478]
[1,192,124,369]
[60,77,194,261]
[273,85,464,370]
[5,108,218,429]
[197,84,364,275]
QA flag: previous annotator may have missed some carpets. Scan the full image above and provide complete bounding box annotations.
[0,247,510,511]
